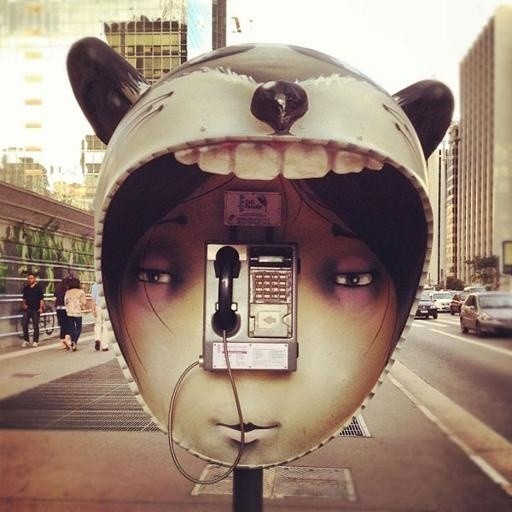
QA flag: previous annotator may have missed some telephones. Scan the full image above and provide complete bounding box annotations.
[212,246,241,337]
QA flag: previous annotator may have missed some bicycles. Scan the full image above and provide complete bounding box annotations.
[17,297,56,340]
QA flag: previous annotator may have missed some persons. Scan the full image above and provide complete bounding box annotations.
[91,282,110,351]
[20,273,44,346]
[54,277,70,341]
[61,277,87,351]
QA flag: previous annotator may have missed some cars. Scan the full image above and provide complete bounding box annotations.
[415,283,489,319]
[460,290,512,337]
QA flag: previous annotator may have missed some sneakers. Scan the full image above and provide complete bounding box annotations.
[60,334,109,353]
[21,340,39,347]
